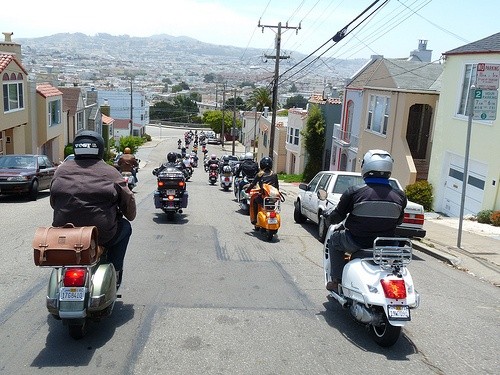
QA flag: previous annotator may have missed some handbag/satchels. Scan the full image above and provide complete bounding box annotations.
[32,223,98,266]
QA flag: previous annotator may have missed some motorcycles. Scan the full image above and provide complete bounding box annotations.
[153,171,189,221]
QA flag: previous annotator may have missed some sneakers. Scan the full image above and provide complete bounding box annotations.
[327,280,339,293]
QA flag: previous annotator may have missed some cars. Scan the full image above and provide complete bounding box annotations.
[113,154,122,167]
[0,154,58,201]
[293,171,427,244]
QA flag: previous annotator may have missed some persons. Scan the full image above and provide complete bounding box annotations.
[236,152,259,198]
[326,149,407,294]
[152,152,188,179]
[176,130,211,181]
[245,157,279,224]
[118,147,138,183]
[206,151,245,193]
[50,130,136,291]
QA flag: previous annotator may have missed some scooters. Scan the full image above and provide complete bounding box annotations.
[317,188,420,348]
[39,246,124,342]
[121,172,137,192]
[167,136,257,215]
[243,176,282,241]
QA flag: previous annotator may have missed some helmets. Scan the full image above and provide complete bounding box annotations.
[360,149,393,180]
[72,130,105,160]
[239,153,246,161]
[185,154,190,159]
[223,155,229,160]
[245,152,253,160]
[167,152,176,163]
[260,157,273,170]
[211,155,216,159]
[124,147,131,154]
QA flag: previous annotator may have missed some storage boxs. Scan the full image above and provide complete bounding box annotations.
[209,164,218,170]
[223,166,232,172]
[159,167,185,187]
